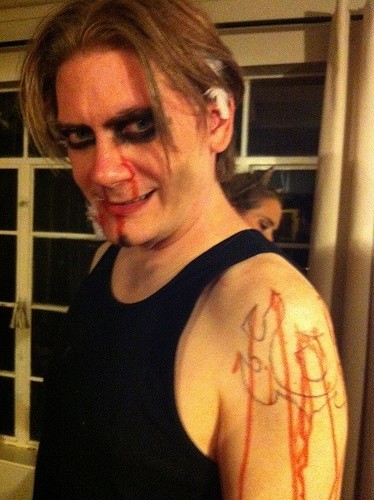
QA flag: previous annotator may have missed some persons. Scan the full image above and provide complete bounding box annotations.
[218,171,283,244]
[20,0,347,500]
